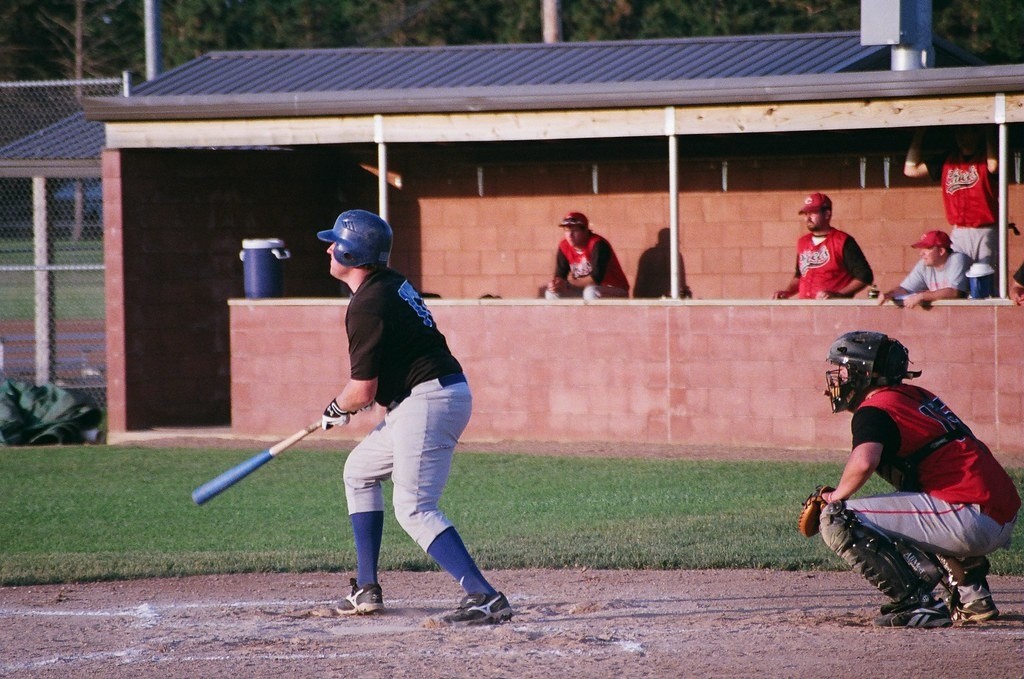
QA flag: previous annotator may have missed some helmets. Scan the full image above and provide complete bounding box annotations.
[825,331,910,414]
[317,210,393,267]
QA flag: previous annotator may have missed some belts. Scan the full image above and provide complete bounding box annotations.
[437,372,466,387]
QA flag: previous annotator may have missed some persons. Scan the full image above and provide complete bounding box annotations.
[1009,262,1024,307]
[317,210,515,627]
[879,229,974,309]
[904,127,999,267]
[769,192,874,300]
[798,331,1021,626]
[545,212,629,298]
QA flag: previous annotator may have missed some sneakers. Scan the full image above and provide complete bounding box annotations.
[954,596,999,622]
[337,577,384,615]
[441,590,513,625]
[874,598,953,627]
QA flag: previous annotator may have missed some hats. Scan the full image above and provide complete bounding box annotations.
[798,193,831,213]
[558,212,589,229]
[912,230,951,248]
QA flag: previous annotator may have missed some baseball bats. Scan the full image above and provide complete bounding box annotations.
[191,419,323,506]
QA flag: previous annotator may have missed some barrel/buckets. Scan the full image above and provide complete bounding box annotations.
[239,238,291,300]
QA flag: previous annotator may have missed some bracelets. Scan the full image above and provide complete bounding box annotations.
[828,493,832,502]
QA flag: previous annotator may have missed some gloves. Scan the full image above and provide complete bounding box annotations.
[321,398,350,430]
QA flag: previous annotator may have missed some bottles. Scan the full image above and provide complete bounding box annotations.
[868,285,880,299]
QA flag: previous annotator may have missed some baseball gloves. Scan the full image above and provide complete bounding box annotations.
[797,484,850,539]
